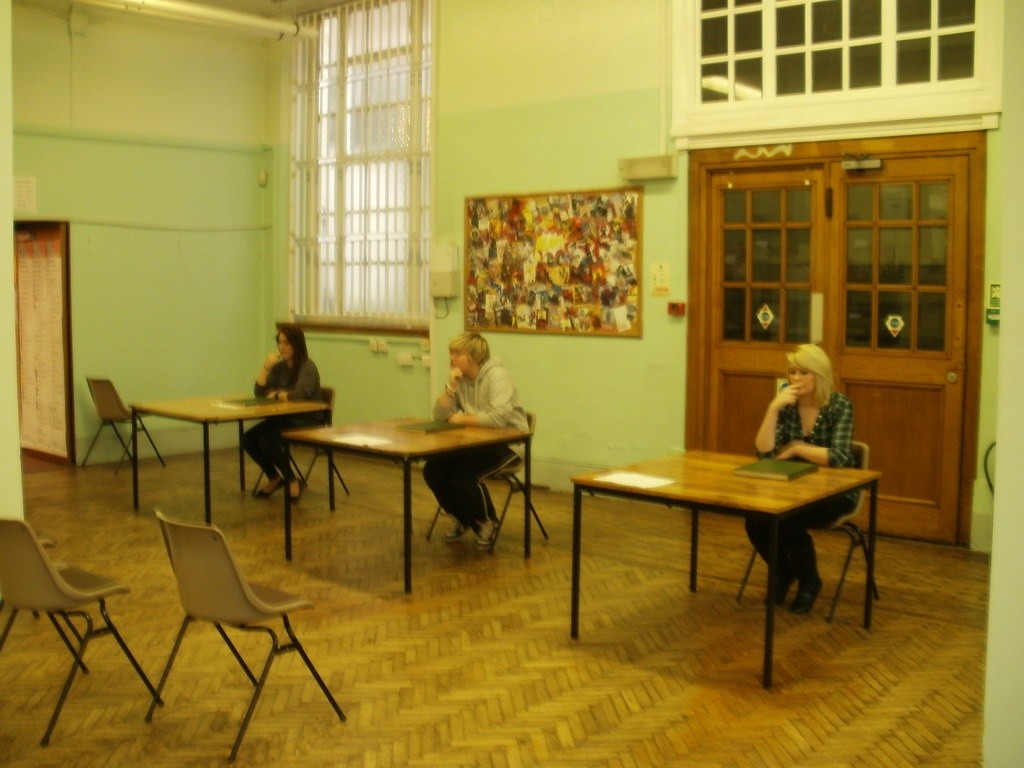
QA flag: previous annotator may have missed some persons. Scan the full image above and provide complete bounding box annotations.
[241,325,323,502]
[422,335,530,550]
[742,344,856,617]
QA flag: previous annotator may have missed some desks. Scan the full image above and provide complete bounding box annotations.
[282,416,534,594]
[570,448,882,691]
[128,393,337,530]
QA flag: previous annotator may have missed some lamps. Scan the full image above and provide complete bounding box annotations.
[80,0,319,43]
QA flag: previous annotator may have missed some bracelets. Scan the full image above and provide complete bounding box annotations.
[263,366,270,371]
[445,384,459,398]
[274,391,281,399]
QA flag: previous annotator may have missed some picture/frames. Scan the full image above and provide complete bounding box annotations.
[462,184,643,339]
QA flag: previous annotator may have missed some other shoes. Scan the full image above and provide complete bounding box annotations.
[291,477,302,505]
[766,568,795,605]
[256,478,285,499]
[475,520,499,550]
[789,576,822,614]
[444,525,470,543]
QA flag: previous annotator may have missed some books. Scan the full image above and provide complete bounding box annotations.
[396,422,465,435]
[212,397,281,408]
[734,458,817,482]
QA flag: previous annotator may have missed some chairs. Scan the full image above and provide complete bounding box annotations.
[736,440,882,624]
[0,517,166,748]
[82,378,168,477]
[251,386,351,504]
[425,413,549,557]
[1,530,55,620]
[143,509,348,765]
